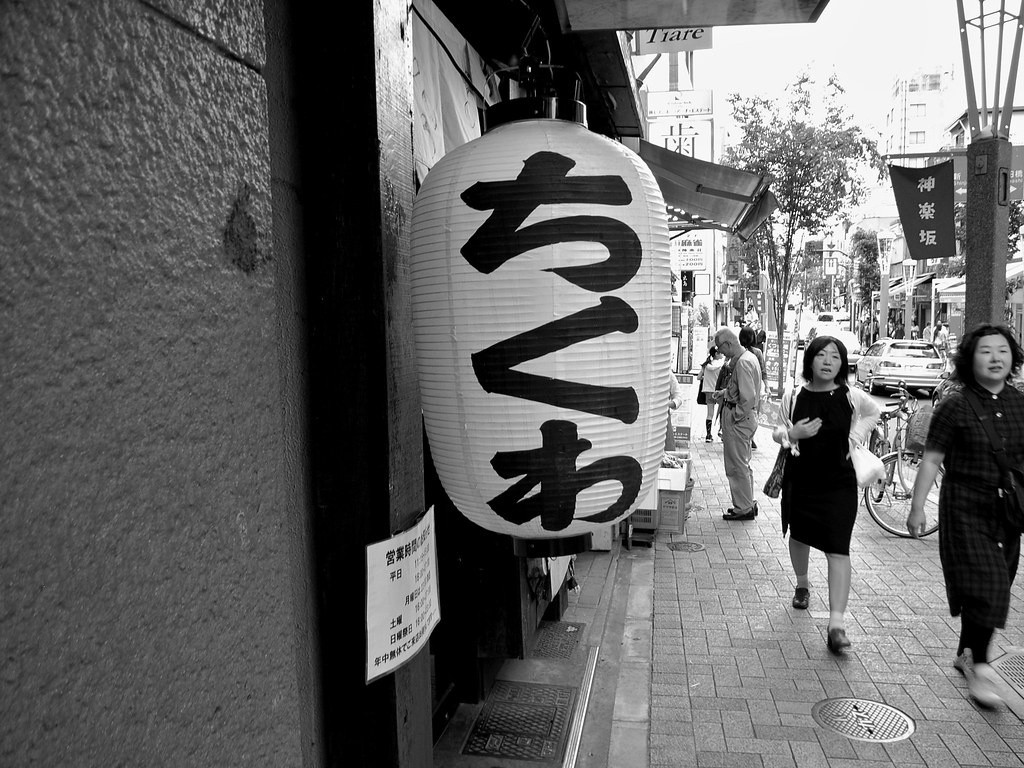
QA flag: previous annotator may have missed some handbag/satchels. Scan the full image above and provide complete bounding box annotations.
[998,462,1024,534]
[848,437,886,488]
[763,469,783,498]
[697,379,716,405]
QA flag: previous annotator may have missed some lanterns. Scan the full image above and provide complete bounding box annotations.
[409,116,672,540]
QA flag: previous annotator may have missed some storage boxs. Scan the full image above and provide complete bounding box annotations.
[630,451,694,535]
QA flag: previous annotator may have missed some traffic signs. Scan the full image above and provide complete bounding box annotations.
[950,144,1024,201]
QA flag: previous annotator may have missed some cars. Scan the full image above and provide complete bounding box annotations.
[798,311,862,372]
[931,371,1024,409]
[854,336,945,399]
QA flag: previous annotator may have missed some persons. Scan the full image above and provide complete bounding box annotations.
[737,321,771,451]
[856,312,951,347]
[772,335,881,650]
[907,325,1024,706]
[698,346,723,443]
[707,326,762,521]
[668,370,683,409]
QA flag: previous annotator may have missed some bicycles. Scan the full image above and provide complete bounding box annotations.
[859,379,946,538]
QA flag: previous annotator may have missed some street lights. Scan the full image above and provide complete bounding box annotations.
[875,229,895,337]
[901,258,918,340]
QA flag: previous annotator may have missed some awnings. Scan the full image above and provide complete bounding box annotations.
[853,276,902,298]
[874,271,935,303]
[628,138,779,245]
[940,260,1024,304]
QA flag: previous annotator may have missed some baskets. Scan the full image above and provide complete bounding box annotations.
[906,412,932,451]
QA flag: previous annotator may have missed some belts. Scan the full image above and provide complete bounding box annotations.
[724,401,736,410]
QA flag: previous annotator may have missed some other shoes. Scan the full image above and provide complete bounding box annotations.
[706,435,713,442]
[718,430,722,437]
[723,512,754,520]
[952,648,1001,708]
[728,504,758,516]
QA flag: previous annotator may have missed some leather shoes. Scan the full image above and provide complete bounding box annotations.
[793,585,810,608]
[827,626,850,647]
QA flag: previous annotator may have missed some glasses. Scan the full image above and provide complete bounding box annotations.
[714,341,731,352]
